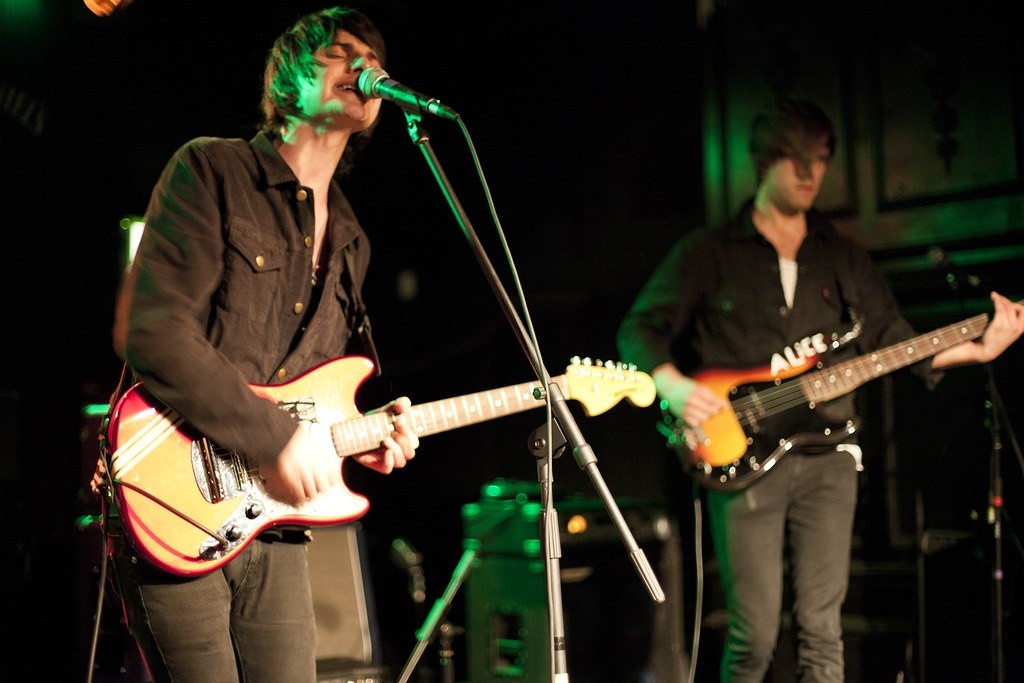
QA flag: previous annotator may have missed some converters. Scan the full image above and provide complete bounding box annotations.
[467,543,684,683]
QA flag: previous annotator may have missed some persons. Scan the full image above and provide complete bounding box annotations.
[617,102,1024,683]
[116,7,421,683]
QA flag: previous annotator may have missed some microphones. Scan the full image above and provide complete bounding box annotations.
[358,66,459,121]
[929,248,981,292]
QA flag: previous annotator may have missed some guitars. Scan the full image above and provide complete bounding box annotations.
[106,353,659,578]
[661,293,1024,493]
